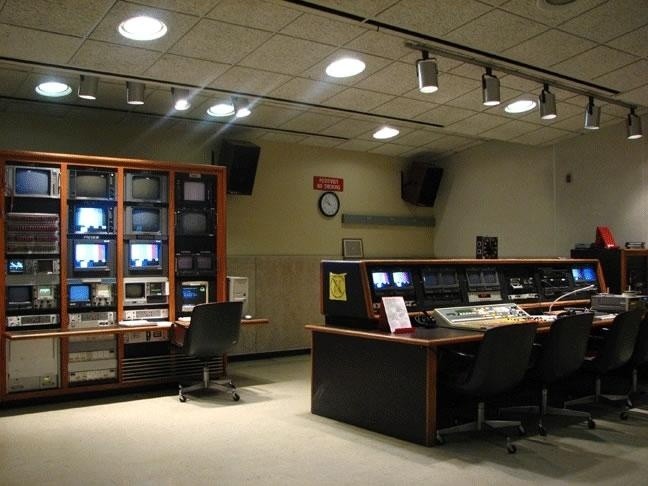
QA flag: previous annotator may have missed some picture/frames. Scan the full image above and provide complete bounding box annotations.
[342,238,365,258]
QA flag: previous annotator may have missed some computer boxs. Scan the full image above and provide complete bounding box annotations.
[227,276,248,318]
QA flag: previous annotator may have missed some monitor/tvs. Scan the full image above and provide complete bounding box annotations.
[175,171,217,206]
[124,206,168,241]
[68,200,117,234]
[4,165,60,199]
[67,168,117,200]
[124,282,146,301]
[124,172,168,203]
[123,240,169,277]
[69,284,91,302]
[175,206,216,238]
[182,281,209,312]
[67,238,117,278]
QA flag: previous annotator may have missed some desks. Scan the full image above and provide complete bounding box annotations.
[298,252,647,448]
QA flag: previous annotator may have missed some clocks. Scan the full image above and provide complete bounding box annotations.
[317,191,341,216]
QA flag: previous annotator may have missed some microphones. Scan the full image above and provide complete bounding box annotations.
[544,284,595,315]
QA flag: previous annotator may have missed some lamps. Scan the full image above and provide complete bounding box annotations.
[75,73,256,120]
[399,39,645,141]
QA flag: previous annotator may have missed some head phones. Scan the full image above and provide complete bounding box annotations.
[414,309,436,329]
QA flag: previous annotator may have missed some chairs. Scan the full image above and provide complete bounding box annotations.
[167,298,243,402]
[437,321,535,455]
[559,307,642,424]
[498,311,598,440]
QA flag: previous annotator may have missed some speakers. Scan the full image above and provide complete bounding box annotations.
[403,161,443,207]
[218,139,260,196]
[476,236,498,259]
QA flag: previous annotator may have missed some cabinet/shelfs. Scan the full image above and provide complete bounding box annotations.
[571,245,647,295]
[0,147,271,406]
